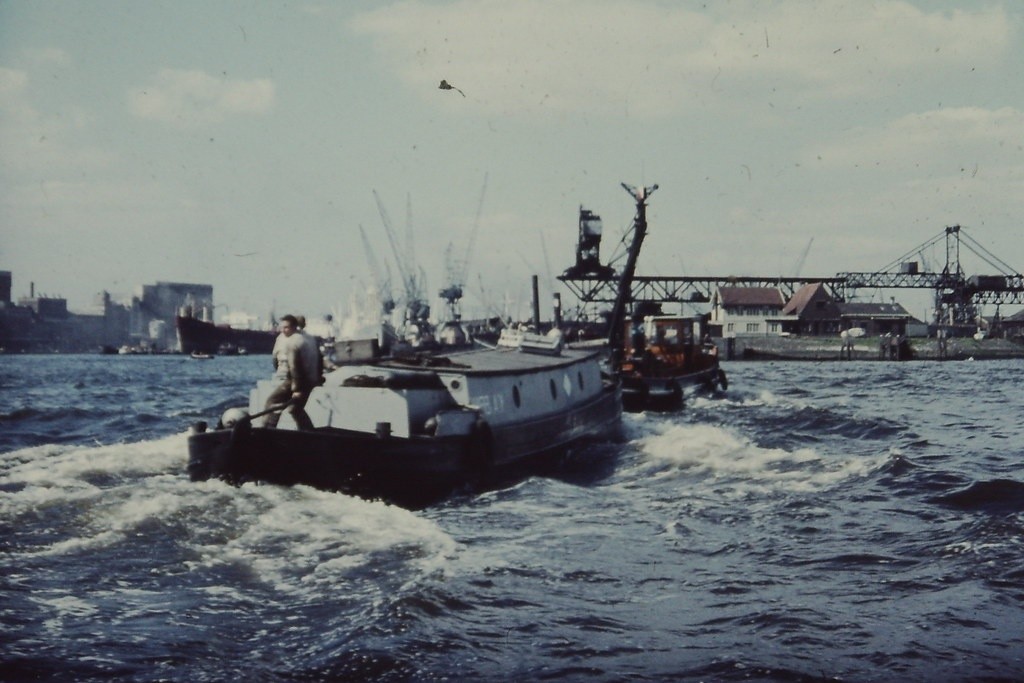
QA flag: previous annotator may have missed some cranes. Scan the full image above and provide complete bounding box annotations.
[836,225,1024,323]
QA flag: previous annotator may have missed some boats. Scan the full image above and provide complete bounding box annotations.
[97,185,732,511]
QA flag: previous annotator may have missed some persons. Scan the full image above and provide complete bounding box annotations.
[271,314,322,393]
[264,317,329,431]
